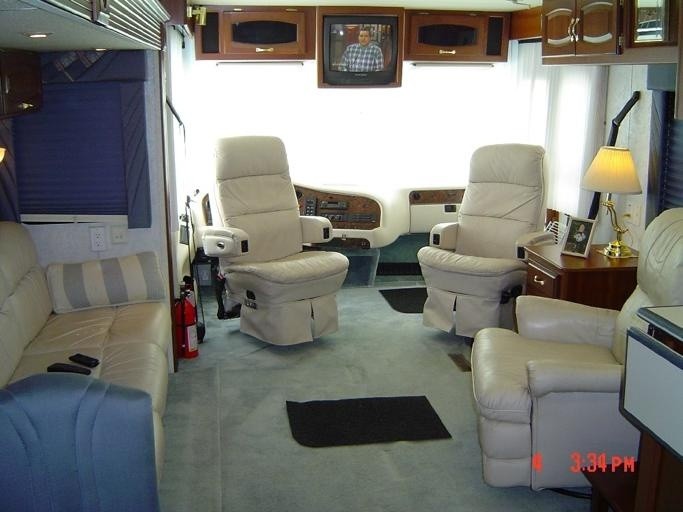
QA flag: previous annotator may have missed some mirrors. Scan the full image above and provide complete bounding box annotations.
[630,0,677,49]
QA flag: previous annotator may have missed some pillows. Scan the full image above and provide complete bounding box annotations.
[44,251,167,313]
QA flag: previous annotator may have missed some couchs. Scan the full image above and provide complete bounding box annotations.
[0,220,170,512]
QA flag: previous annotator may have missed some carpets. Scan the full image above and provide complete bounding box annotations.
[284,395,453,447]
[378,287,427,313]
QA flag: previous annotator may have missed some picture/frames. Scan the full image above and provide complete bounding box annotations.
[560,217,597,258]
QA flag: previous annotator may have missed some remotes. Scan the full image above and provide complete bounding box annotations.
[69,353,100,368]
[47,363,91,375]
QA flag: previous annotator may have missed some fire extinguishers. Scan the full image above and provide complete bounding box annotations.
[174,291,198,358]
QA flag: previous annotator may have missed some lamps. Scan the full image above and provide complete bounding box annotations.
[580,146,643,259]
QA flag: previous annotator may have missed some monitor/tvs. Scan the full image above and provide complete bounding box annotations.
[323,14,399,85]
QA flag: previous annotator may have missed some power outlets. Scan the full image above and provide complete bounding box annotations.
[88,226,107,252]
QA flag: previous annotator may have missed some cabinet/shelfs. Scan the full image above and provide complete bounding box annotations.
[521,244,639,311]
[404,9,511,61]
[0,50,44,119]
[580,462,639,512]
[617,303,682,512]
[542,0,628,65]
[194,7,315,60]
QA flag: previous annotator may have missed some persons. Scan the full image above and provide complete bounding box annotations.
[338,28,384,71]
[571,223,586,253]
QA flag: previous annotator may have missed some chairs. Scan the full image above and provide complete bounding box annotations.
[201,136,350,347]
[470,208,682,490]
[416,143,554,339]
[1,372,159,512]
